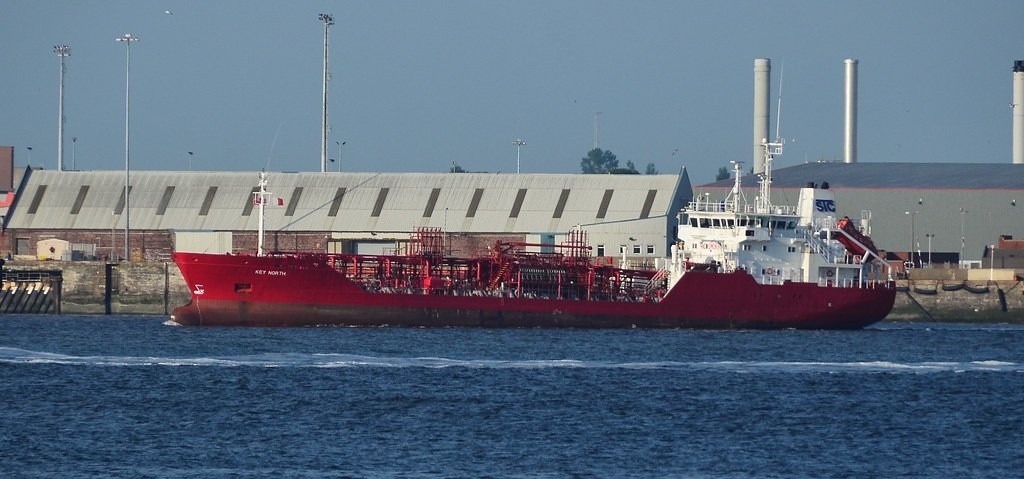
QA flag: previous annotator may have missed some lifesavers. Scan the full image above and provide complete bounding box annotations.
[826,270,834,277]
[767,268,773,275]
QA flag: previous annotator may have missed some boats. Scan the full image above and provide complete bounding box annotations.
[163,44,900,330]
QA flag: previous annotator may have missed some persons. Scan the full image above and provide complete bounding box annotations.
[676,240,684,249]
[658,291,662,301]
[7,252,11,260]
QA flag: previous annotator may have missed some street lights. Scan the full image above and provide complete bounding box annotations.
[905,211,920,269]
[513,138,527,174]
[117,32,139,262]
[319,13,335,172]
[990,245,995,280]
[54,44,73,170]
[926,234,935,268]
